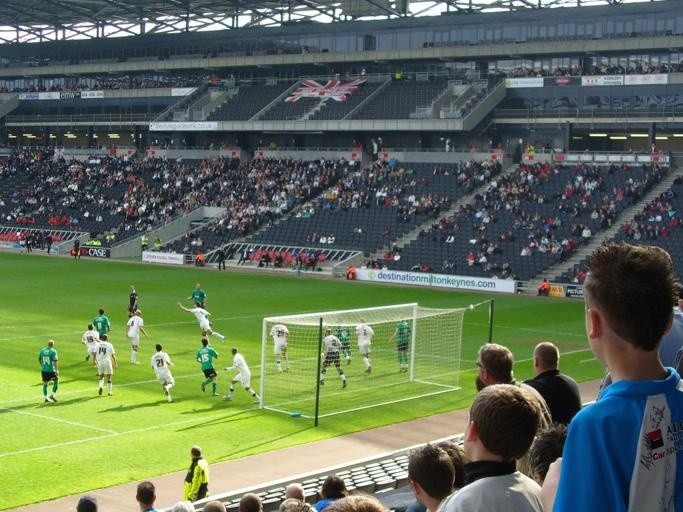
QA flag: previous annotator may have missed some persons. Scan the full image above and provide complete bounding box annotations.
[77,494,99,512]
[334,317,353,365]
[268,319,290,374]
[85,308,110,361]
[176,301,226,347]
[540,240,682,512]
[0,72,219,92]
[354,317,374,374]
[404,341,583,510]
[150,343,176,402]
[194,337,221,396]
[0,137,682,296]
[240,493,263,512]
[386,314,411,373]
[510,60,682,77]
[175,500,226,512]
[280,473,390,512]
[136,480,158,512]
[36,340,60,403]
[658,279,683,369]
[81,322,102,376]
[91,333,118,396]
[221,348,260,403]
[186,284,212,326]
[125,284,138,321]
[124,310,149,364]
[183,446,210,501]
[320,329,348,389]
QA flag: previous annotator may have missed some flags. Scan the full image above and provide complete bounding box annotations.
[285,79,367,103]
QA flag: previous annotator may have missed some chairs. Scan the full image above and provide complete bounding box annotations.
[2,149,681,294]
[206,74,447,122]
[223,450,412,512]
